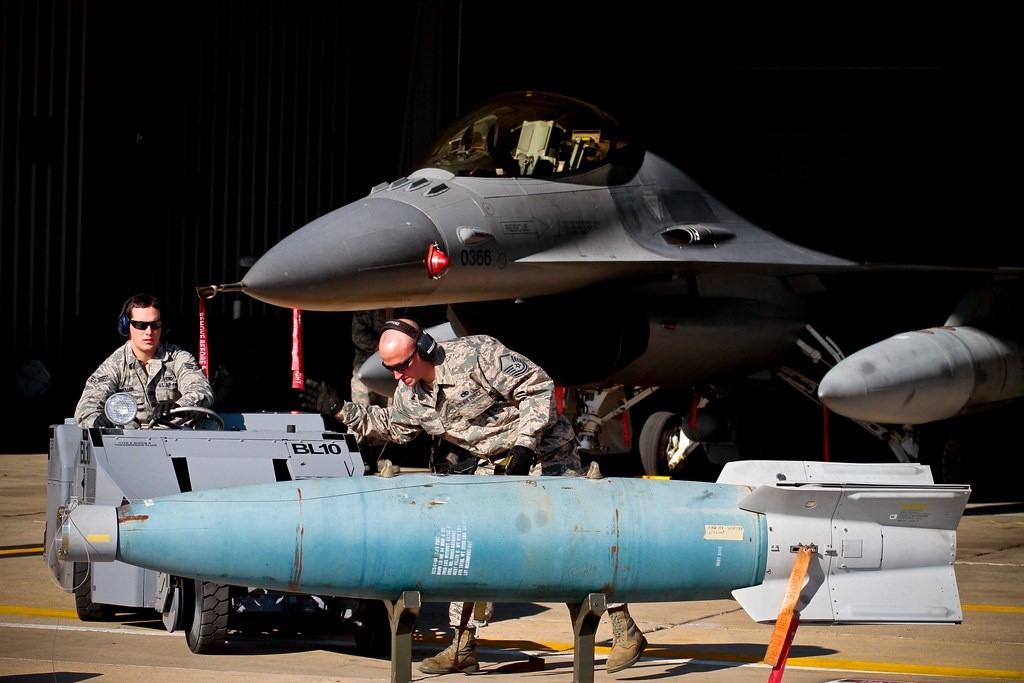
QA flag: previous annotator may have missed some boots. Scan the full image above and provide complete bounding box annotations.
[418,627,479,673]
[606,602,648,673]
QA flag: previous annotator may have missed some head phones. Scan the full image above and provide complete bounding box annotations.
[118,293,169,337]
[378,320,437,361]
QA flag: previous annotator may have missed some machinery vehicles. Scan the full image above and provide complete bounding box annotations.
[47,402,425,652]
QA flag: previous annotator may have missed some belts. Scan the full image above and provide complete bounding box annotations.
[477,447,510,468]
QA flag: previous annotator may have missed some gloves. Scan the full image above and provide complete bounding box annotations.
[93,414,116,428]
[148,401,185,424]
[501,445,535,475]
[299,379,344,419]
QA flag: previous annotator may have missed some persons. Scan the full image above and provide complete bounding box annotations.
[74,294,215,431]
[299,317,648,675]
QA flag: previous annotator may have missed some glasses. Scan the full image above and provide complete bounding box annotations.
[130,318,164,330]
[381,347,420,372]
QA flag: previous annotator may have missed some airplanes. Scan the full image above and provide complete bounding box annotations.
[197,89,1022,485]
